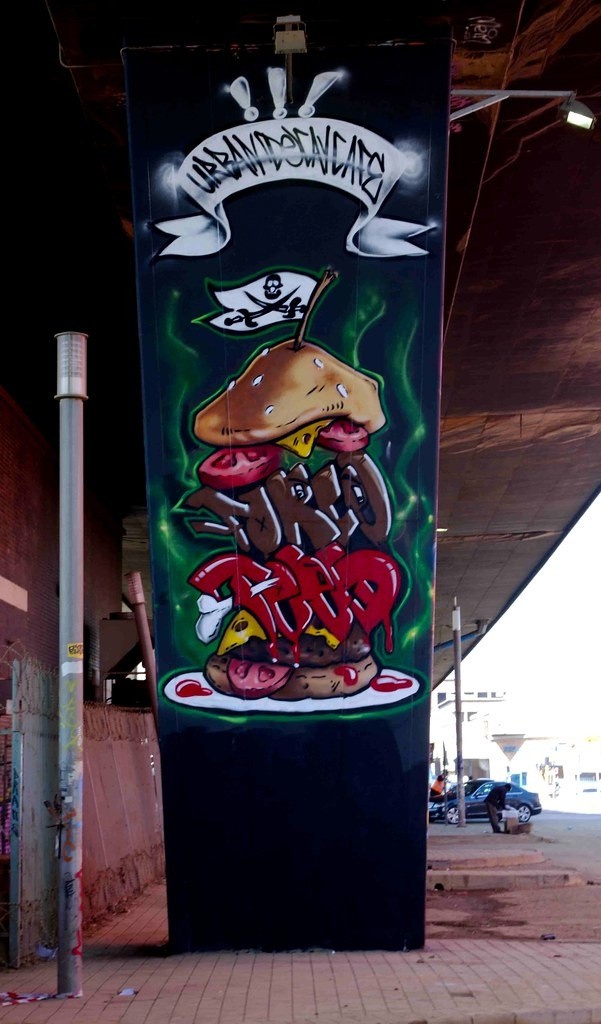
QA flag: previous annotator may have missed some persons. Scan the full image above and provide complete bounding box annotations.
[468,775,473,780]
[430,770,449,796]
[483,783,512,833]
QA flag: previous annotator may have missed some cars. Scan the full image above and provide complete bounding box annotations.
[429,780,542,824]
